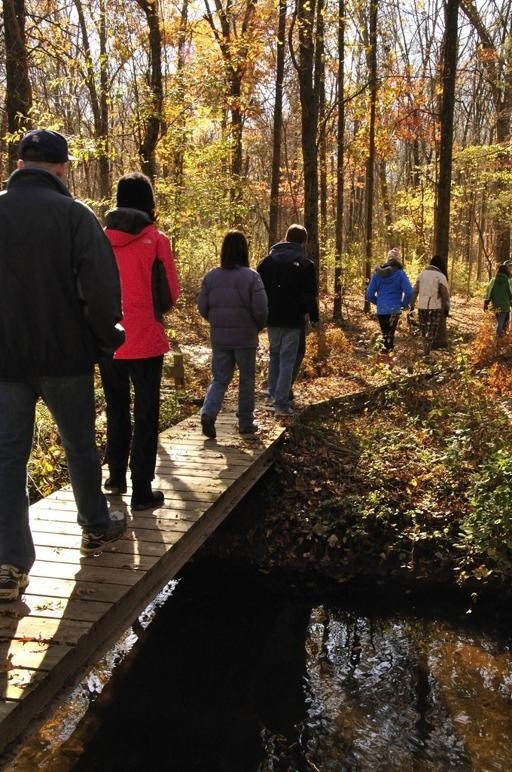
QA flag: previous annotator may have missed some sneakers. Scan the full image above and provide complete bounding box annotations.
[80,509,126,556]
[274,407,295,417]
[104,477,127,493]
[0,563,29,599]
[200,414,217,438]
[131,491,164,511]
[239,423,258,435]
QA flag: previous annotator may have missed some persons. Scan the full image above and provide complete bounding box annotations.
[366,247,413,353]
[482,264,512,338]
[288,312,310,402]
[96,172,184,515]
[502,261,511,331]
[0,125,135,605]
[193,228,266,443]
[406,252,452,365]
[253,220,324,421]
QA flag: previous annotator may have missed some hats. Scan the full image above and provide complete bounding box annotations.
[19,128,83,163]
[385,247,400,263]
